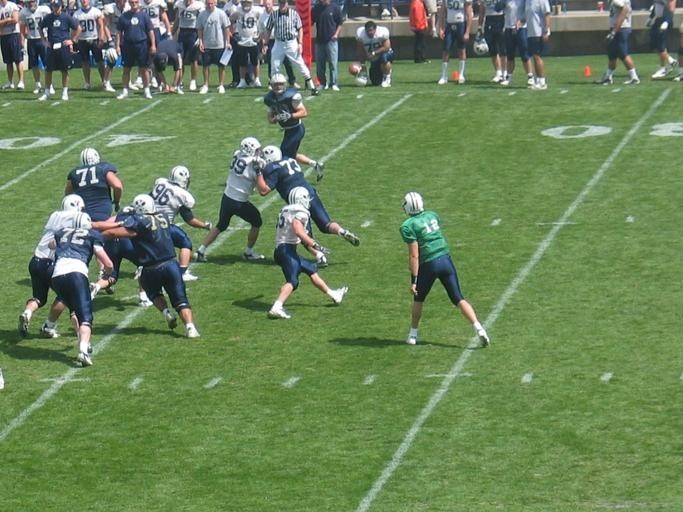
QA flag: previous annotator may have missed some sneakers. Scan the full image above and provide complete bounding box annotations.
[243,252,265,260]
[527,75,547,90]
[315,161,324,181]
[88,283,98,301]
[229,80,262,88]
[438,78,448,84]
[340,230,360,246]
[490,75,512,86]
[40,323,61,338]
[269,308,291,319]
[406,334,417,345]
[652,58,683,81]
[333,286,348,303]
[78,352,93,366]
[164,310,178,328]
[193,251,207,261]
[187,326,200,337]
[82,83,91,90]
[477,328,489,345]
[381,74,391,87]
[218,84,225,93]
[459,78,465,83]
[61,86,69,100]
[594,72,614,85]
[103,79,184,99]
[19,313,29,338]
[290,83,301,89]
[624,76,640,85]
[312,255,328,268]
[181,272,198,281]
[1,80,55,101]
[139,298,153,306]
[189,79,197,91]
[312,84,339,96]
[199,85,208,94]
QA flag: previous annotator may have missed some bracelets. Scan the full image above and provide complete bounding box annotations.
[291,114,293,118]
[411,274,418,284]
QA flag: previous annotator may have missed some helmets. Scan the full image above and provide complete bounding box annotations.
[403,191,424,214]
[239,136,282,164]
[352,76,368,87]
[271,73,286,94]
[169,165,189,189]
[133,194,156,214]
[288,186,310,210]
[81,147,101,166]
[474,39,489,56]
[61,194,93,230]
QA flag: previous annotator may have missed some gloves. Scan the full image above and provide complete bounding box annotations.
[603,31,616,43]
[251,158,264,175]
[203,221,212,229]
[273,111,292,123]
[313,242,330,254]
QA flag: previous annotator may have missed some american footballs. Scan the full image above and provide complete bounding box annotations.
[349,64,360,75]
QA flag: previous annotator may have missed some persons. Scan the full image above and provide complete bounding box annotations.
[16,146,212,367]
[593,0,640,85]
[193,137,360,266]
[0,0,344,95]
[355,20,394,88]
[408,0,552,91]
[400,190,491,347]
[263,76,324,181]
[643,0,683,82]
[268,186,348,319]
